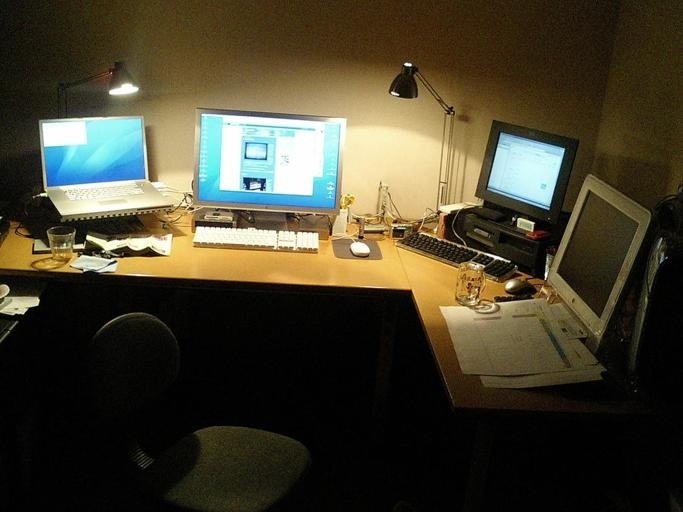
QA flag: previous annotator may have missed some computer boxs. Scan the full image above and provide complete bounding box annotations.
[448,206,572,279]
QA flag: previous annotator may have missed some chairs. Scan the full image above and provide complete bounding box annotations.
[77,311,322,509]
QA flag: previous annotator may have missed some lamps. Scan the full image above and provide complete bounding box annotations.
[55,62,142,116]
[380,58,458,224]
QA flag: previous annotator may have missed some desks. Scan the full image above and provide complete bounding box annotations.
[0,204,668,417]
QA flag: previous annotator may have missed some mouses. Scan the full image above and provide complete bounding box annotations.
[350,241,370,257]
[505,279,537,295]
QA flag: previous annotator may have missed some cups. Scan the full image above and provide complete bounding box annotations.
[455,260,484,307]
[47,227,78,261]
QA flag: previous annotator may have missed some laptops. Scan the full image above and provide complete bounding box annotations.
[39,115,172,222]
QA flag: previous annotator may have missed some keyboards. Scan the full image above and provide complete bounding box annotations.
[394,230,519,284]
[192,226,320,253]
[494,294,535,302]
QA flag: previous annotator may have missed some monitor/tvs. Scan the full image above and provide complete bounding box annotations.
[193,108,347,239]
[537,173,653,353]
[474,120,579,235]
